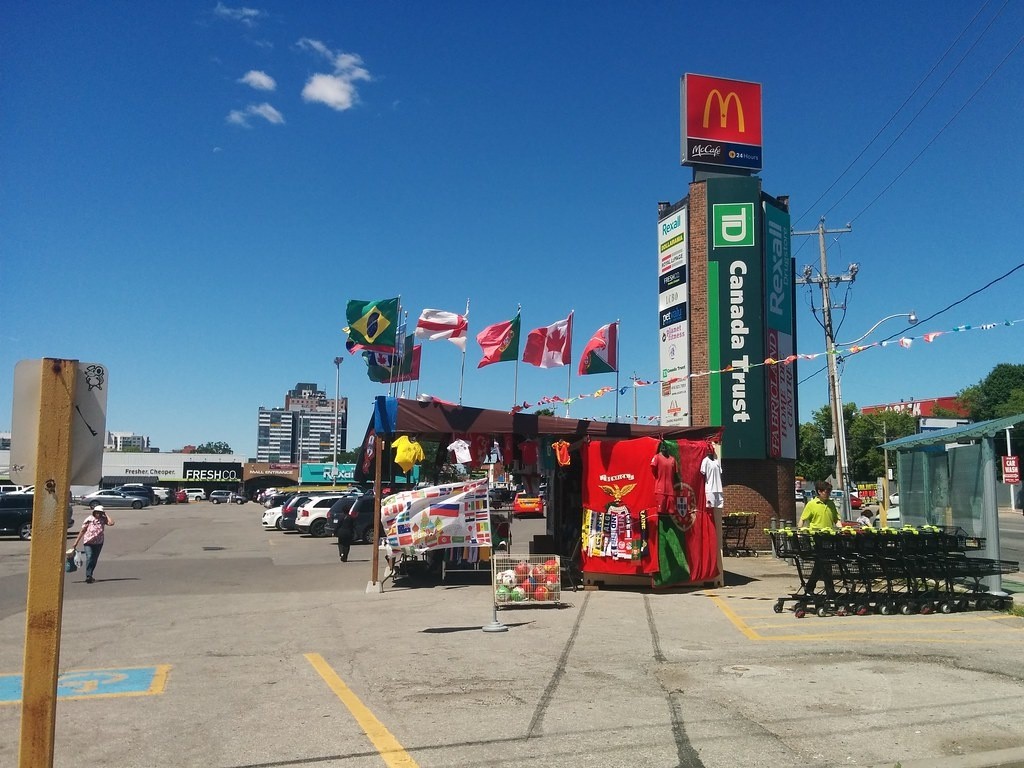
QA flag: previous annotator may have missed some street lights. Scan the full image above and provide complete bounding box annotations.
[823,311,918,527]
[332,355,344,489]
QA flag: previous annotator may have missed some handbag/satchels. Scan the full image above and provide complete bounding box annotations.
[74,551,81,568]
[66,556,79,572]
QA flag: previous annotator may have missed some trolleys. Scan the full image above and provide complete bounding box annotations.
[720,510,760,558]
[766,524,1021,619]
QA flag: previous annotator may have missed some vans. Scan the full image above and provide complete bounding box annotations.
[179,487,207,502]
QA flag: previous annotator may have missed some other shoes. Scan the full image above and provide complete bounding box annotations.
[340,553,345,562]
[85,576,96,584]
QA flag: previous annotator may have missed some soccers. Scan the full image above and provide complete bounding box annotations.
[496,559,559,601]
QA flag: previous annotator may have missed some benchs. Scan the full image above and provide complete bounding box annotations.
[842,519,867,536]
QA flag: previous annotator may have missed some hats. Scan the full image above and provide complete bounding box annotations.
[92,505,105,512]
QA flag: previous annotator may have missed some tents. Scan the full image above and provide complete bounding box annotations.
[353,396,722,584]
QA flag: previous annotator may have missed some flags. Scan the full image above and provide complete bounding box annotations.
[380,478,492,558]
[522,313,573,369]
[342,297,420,383]
[476,312,519,368]
[669,321,1014,405]
[511,369,658,421]
[577,321,617,376]
[414,303,469,351]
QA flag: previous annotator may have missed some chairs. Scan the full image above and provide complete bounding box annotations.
[559,536,583,593]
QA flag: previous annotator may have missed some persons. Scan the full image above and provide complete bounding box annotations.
[649,446,681,513]
[332,504,354,560]
[386,555,401,578]
[799,481,844,600]
[856,510,882,528]
[73,506,114,583]
[701,452,723,510]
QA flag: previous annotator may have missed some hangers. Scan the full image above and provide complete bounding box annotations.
[455,434,460,441]
[525,436,532,442]
[558,438,564,442]
[409,433,417,442]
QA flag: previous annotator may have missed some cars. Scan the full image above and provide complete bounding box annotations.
[514,491,545,518]
[324,493,392,545]
[112,482,172,506]
[537,482,548,497]
[795,487,901,522]
[253,487,365,538]
[0,475,75,541]
[174,491,189,503]
[80,489,150,510]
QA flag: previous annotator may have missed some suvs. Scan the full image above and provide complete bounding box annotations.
[209,489,249,505]
